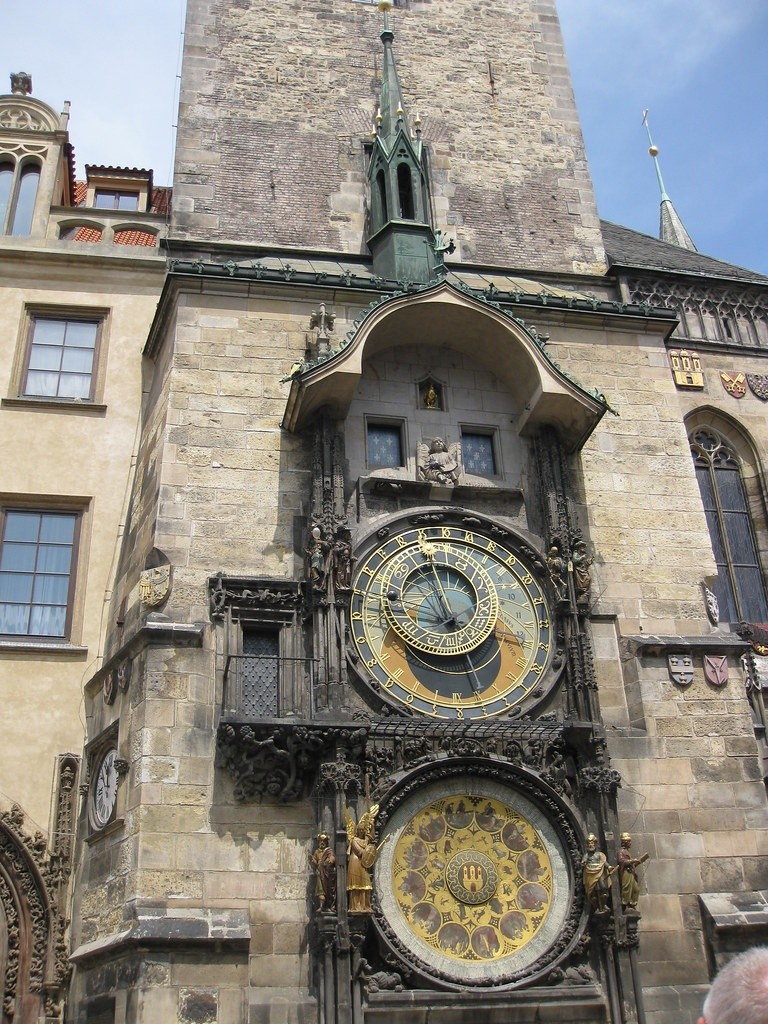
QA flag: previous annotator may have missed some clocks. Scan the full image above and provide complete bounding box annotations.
[350,527,552,720]
[95,748,118,823]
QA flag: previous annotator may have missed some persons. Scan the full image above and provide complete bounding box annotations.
[307,805,392,914]
[416,435,461,486]
[580,832,649,916]
[548,961,596,985]
[547,541,596,600]
[369,971,405,992]
[302,528,359,595]
[697,945,768,1024]
